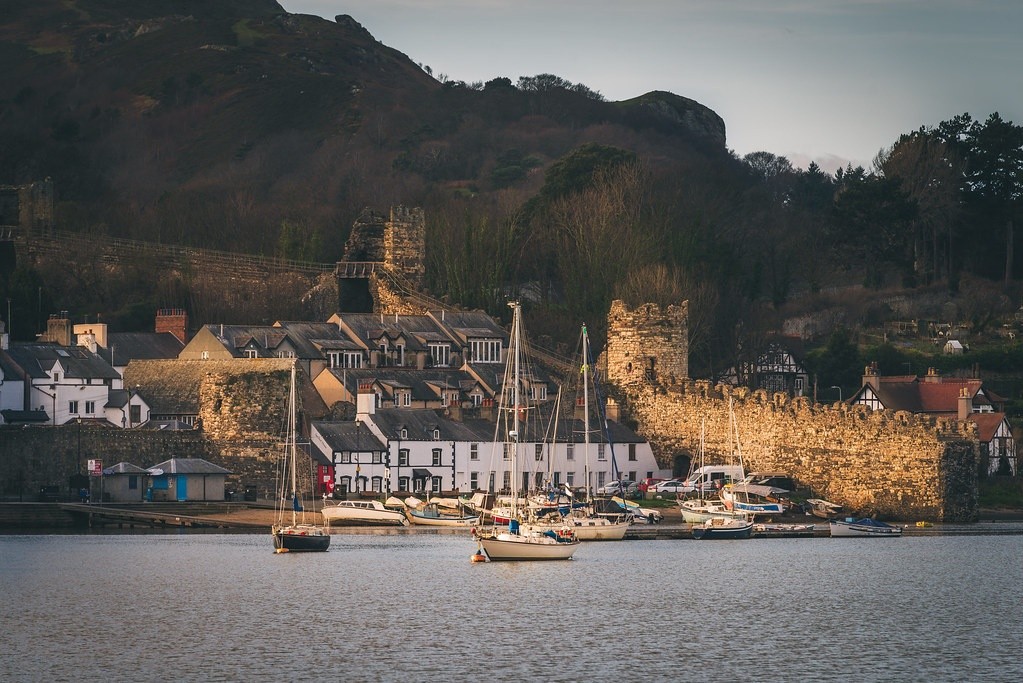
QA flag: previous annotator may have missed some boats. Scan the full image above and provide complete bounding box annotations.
[321,384,663,527]
[828,518,903,538]
[717,487,785,516]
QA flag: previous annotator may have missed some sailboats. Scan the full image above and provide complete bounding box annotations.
[675,418,748,523]
[689,395,755,541]
[470,301,580,562]
[520,320,629,541]
[270,357,331,552]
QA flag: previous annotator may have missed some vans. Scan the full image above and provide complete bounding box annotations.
[684,465,745,493]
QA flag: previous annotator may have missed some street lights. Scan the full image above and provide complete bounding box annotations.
[76,415,81,473]
[831,386,842,402]
[355,418,361,492]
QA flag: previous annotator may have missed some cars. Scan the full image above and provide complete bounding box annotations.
[646,480,700,494]
[596,477,666,497]
[734,471,790,486]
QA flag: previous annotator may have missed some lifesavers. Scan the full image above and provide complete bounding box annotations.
[287,531,295,534]
[298,530,309,535]
[559,526,572,538]
